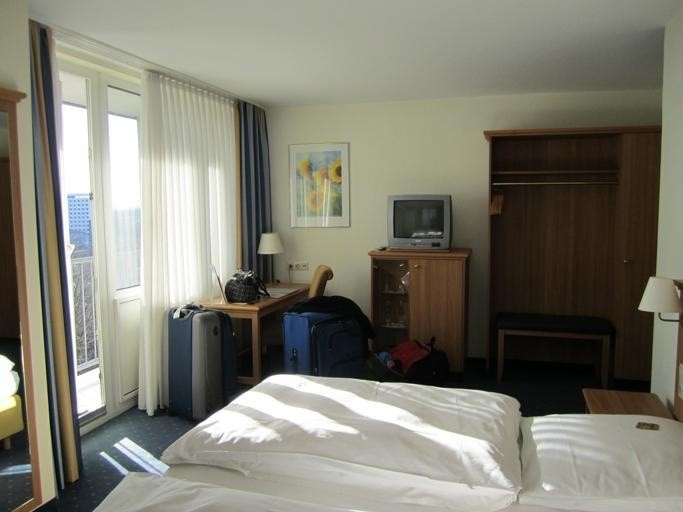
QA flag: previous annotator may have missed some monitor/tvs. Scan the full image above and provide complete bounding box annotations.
[386,194,452,252]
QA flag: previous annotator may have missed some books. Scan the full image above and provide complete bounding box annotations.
[259,286,299,300]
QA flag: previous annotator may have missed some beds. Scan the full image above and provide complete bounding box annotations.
[0,394,25,450]
[92,374,683,512]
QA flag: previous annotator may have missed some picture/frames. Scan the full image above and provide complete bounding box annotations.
[288,143,351,228]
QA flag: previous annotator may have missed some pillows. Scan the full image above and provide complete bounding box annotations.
[521,414,682,511]
[161,373,522,512]
[0,354,16,378]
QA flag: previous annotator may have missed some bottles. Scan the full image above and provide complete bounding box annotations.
[376,347,395,368]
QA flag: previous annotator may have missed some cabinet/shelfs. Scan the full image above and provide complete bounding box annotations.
[368,247,472,373]
[484,123,661,381]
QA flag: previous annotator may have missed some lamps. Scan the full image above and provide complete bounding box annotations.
[257,232,284,284]
[637,277,683,327]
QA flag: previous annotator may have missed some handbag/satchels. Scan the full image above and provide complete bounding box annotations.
[371,333,449,383]
[225,271,270,303]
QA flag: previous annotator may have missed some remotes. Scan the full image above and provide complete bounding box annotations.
[378,245,386,250]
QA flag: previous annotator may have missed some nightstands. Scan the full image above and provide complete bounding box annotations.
[582,387,674,420]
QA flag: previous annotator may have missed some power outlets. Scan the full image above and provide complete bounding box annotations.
[285,257,310,272]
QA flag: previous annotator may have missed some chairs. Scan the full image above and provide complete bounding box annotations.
[308,264,333,299]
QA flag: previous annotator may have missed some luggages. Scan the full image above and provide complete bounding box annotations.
[166,304,236,421]
[283,311,367,379]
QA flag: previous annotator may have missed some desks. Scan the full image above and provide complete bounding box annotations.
[195,283,311,387]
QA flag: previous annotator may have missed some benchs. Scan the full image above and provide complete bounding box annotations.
[497,312,614,391]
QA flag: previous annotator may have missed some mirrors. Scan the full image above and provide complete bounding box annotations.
[0,88,43,511]
